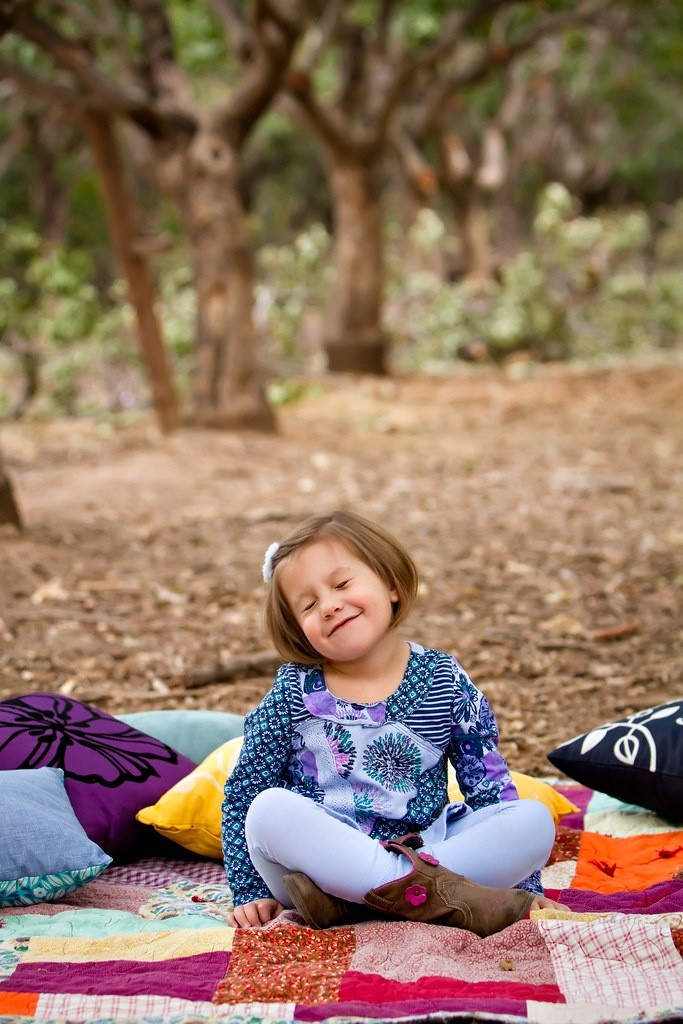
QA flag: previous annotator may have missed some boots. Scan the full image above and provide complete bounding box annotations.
[282,833,423,931]
[363,839,535,940]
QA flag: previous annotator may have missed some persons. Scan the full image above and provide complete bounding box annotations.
[220,511,573,939]
